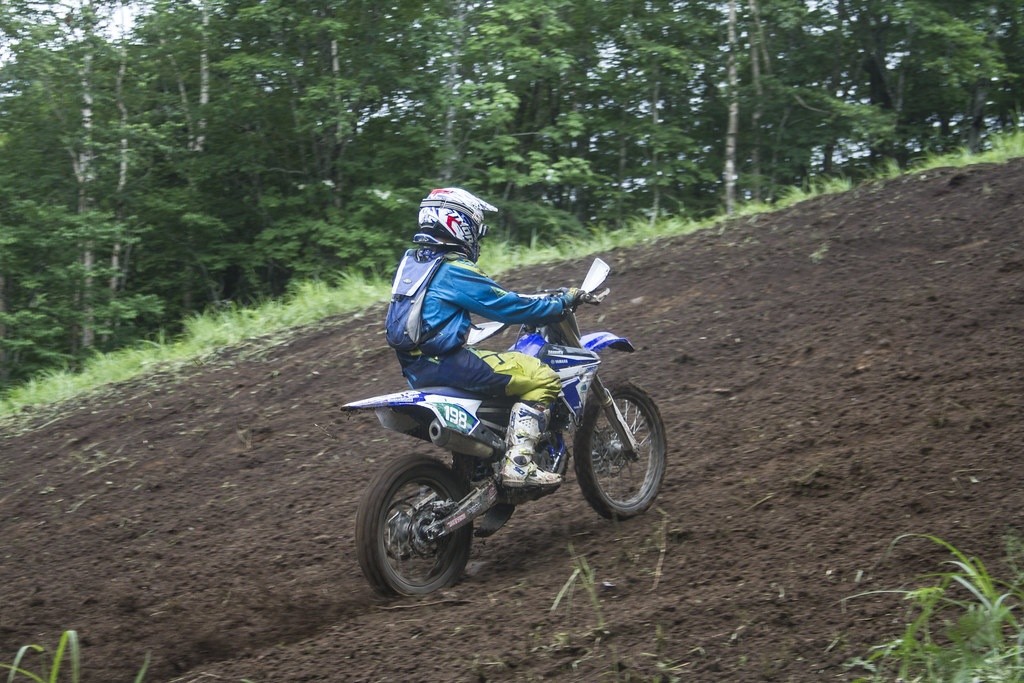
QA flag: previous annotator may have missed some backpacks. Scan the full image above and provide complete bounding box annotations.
[385,248,453,350]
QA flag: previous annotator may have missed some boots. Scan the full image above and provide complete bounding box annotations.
[502,402,563,487]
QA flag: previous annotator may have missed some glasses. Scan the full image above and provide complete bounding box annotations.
[475,223,489,241]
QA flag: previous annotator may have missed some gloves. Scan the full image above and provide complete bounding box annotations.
[560,287,585,309]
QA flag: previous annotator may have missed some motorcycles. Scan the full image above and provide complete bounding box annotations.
[339,256,669,603]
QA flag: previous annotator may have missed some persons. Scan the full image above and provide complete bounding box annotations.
[386,187,586,487]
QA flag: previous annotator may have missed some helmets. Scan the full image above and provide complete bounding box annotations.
[418,187,498,264]
